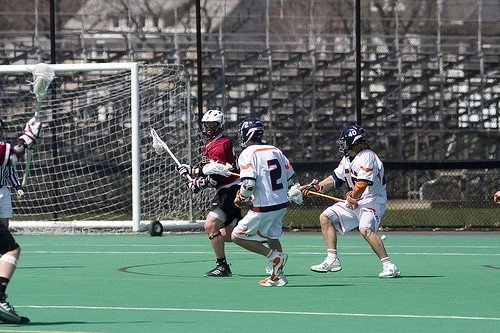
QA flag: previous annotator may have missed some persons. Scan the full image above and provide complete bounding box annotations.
[297,124,400,279]
[173,109,276,277]
[230,118,297,287]
[0,116,41,325]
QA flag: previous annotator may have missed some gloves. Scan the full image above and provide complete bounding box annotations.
[185,176,205,194]
[346,191,364,209]
[299,178,323,199]
[17,118,42,149]
[177,164,193,178]
[233,190,253,210]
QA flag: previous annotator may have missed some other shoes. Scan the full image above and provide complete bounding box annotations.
[0,302,31,325]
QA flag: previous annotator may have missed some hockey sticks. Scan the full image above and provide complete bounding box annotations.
[151,127,194,181]
[202,163,346,202]
[287,182,304,205]
[21,63,56,187]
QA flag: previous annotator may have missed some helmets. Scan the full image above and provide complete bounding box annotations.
[200,109,226,139]
[336,125,367,157]
[237,117,264,149]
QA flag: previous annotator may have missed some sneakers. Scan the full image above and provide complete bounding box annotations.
[266,251,289,280]
[378,263,401,278]
[203,261,233,278]
[258,266,289,287]
[310,256,343,273]
